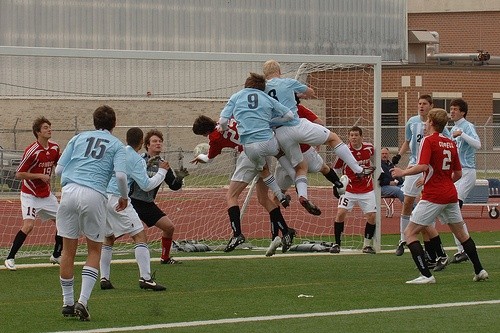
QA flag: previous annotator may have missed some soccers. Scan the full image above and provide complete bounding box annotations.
[192,143,215,164]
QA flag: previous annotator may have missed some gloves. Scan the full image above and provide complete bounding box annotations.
[392,153,401,165]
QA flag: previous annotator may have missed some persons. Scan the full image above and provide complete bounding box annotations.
[192,60,376,258]
[4,117,64,271]
[329,126,376,254]
[389,94,489,285]
[129,128,190,265]
[99,127,169,290]
[381,147,416,210]
[55,105,128,321]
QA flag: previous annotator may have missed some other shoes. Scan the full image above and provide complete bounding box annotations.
[265,237,284,256]
[280,195,292,209]
[337,175,349,196]
[355,165,376,180]
[299,196,321,216]
[62,304,77,317]
[74,301,91,321]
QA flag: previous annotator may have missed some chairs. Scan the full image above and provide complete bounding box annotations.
[480,178,500,217]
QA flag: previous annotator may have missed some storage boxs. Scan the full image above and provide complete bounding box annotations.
[464,179,489,204]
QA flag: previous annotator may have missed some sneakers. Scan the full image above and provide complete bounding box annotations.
[223,233,246,251]
[430,254,449,272]
[100,277,115,289]
[4,258,17,271]
[395,239,407,256]
[139,270,168,291]
[449,250,470,264]
[161,258,183,264]
[329,243,342,253]
[282,228,298,253]
[405,274,436,286]
[471,268,489,282]
[50,255,62,265]
[362,246,376,254]
[428,260,437,268]
